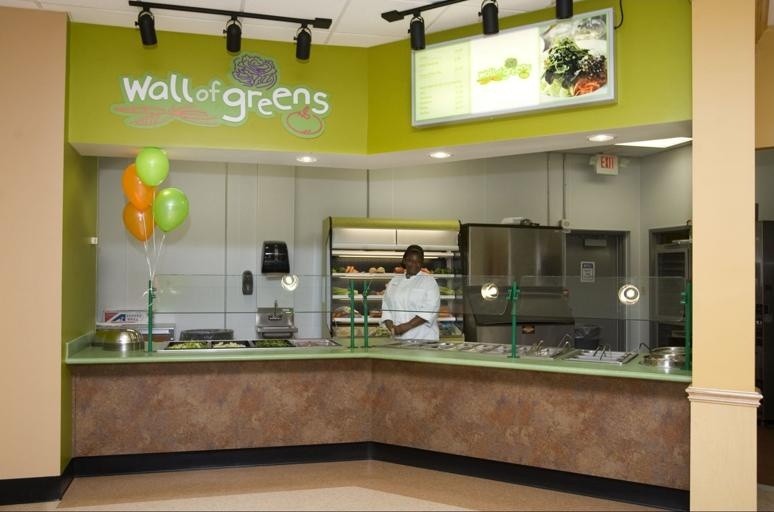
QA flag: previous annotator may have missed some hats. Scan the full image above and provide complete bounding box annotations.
[401,244,425,268]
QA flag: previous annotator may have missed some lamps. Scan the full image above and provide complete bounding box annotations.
[134,3,158,46]
[556,0,574,19]
[223,14,244,54]
[478,1,498,35]
[293,22,312,60]
[406,12,427,50]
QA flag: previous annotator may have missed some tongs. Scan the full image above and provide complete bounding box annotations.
[592,341,612,361]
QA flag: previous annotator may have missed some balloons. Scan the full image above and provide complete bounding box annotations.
[152,187,190,232]
[134,147,169,188]
[123,202,154,240]
[123,163,158,213]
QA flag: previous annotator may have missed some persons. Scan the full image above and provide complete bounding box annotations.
[379,243,439,339]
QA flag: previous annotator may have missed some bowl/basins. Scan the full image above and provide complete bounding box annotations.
[540,37,609,100]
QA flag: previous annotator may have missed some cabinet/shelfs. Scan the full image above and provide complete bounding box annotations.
[462,223,567,290]
[320,216,464,344]
[652,240,692,349]
